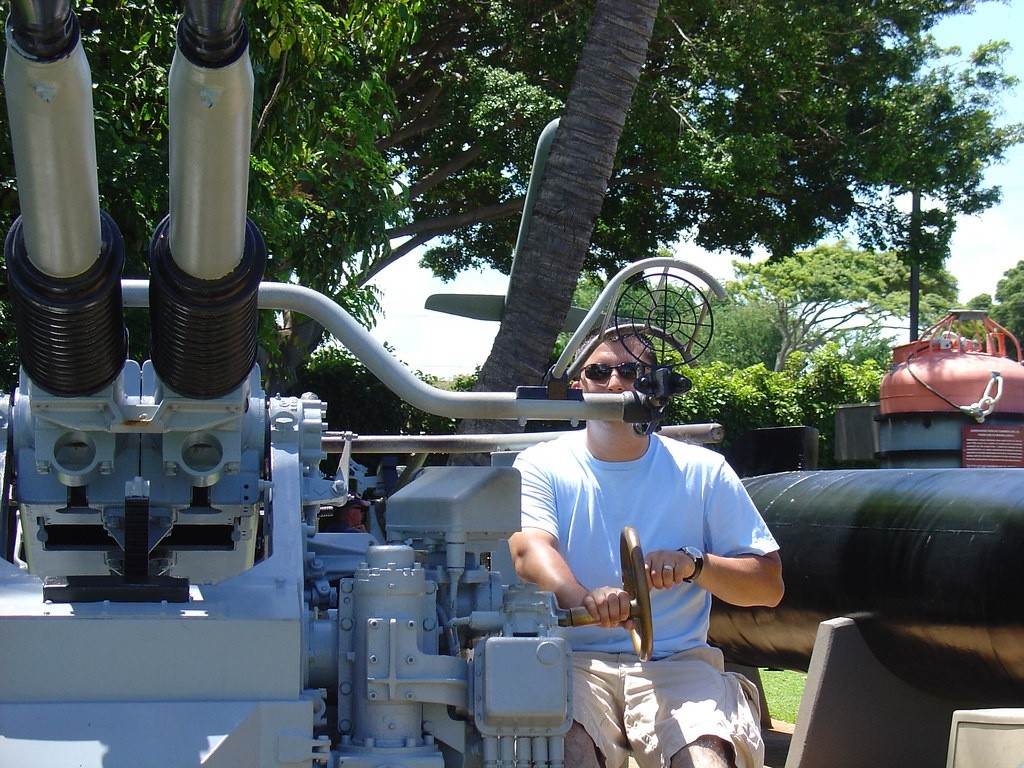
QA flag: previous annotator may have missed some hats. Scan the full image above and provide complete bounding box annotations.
[333,492,371,508]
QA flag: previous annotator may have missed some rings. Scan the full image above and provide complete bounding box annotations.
[663,565,673,570]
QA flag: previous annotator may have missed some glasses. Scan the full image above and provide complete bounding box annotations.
[353,507,364,513]
[578,362,640,380]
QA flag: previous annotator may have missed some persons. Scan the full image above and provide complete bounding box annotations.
[321,499,371,532]
[507,325,784,768]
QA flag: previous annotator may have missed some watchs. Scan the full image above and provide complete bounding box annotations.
[676,546,704,583]
